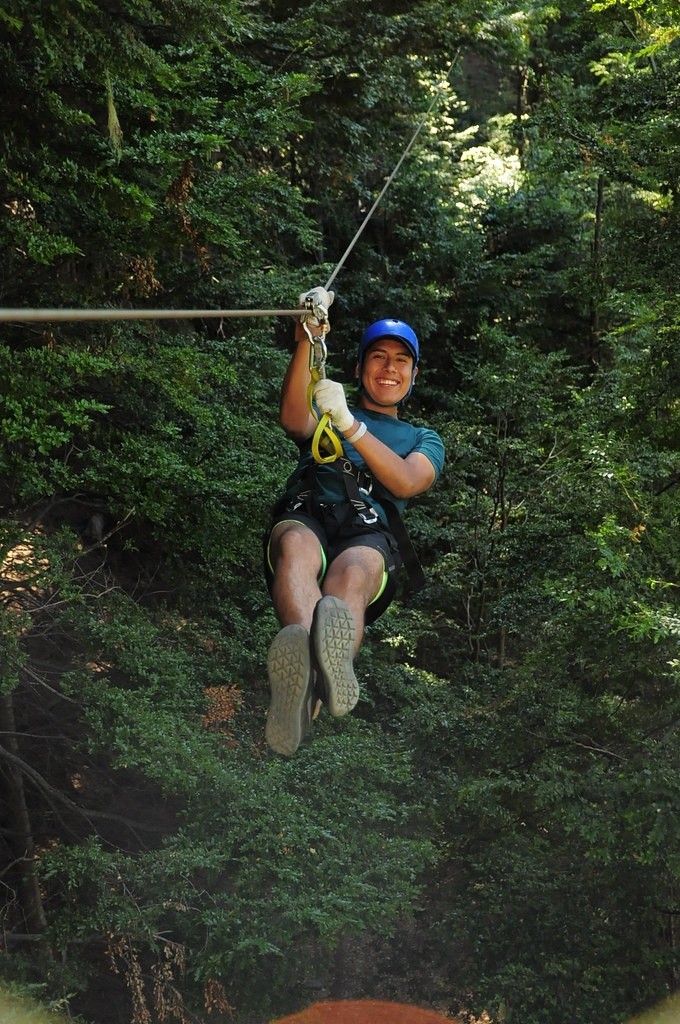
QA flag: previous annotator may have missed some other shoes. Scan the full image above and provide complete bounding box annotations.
[311,595,360,718]
[263,624,313,756]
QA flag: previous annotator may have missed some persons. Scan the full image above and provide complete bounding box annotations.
[262,286,445,757]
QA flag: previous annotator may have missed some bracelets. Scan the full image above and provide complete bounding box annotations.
[343,422,367,444]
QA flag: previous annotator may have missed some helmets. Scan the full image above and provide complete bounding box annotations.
[357,318,420,368]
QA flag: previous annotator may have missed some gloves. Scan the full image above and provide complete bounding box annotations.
[298,287,336,326]
[311,378,356,432]
